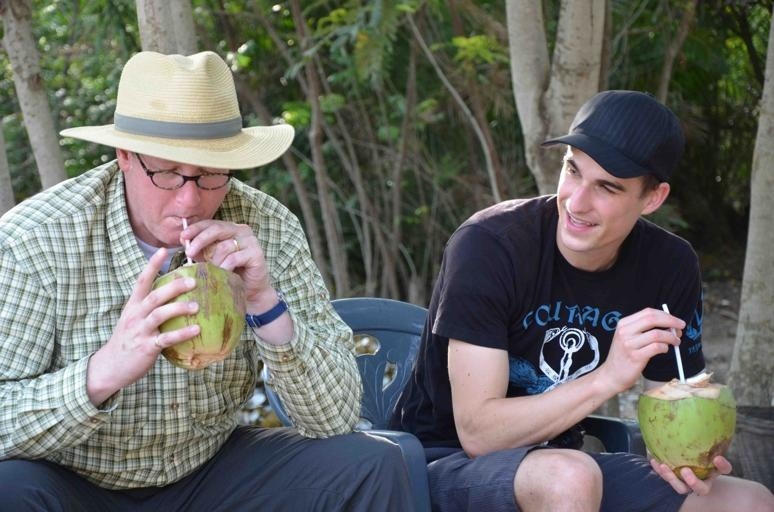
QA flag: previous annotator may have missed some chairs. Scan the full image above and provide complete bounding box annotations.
[264,297,646,457]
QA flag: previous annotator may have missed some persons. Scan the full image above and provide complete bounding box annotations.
[0,50,416,512]
[392,88,773,512]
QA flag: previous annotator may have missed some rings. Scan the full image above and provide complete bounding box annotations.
[233,236,240,250]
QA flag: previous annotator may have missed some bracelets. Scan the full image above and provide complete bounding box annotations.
[245,288,289,328]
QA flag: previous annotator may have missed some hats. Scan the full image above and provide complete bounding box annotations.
[541,89,688,182]
[60,51,296,170]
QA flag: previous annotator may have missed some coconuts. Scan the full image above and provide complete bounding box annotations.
[145,264,246,372]
[637,372,737,482]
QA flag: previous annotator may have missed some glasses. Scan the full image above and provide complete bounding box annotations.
[136,152,234,190]
